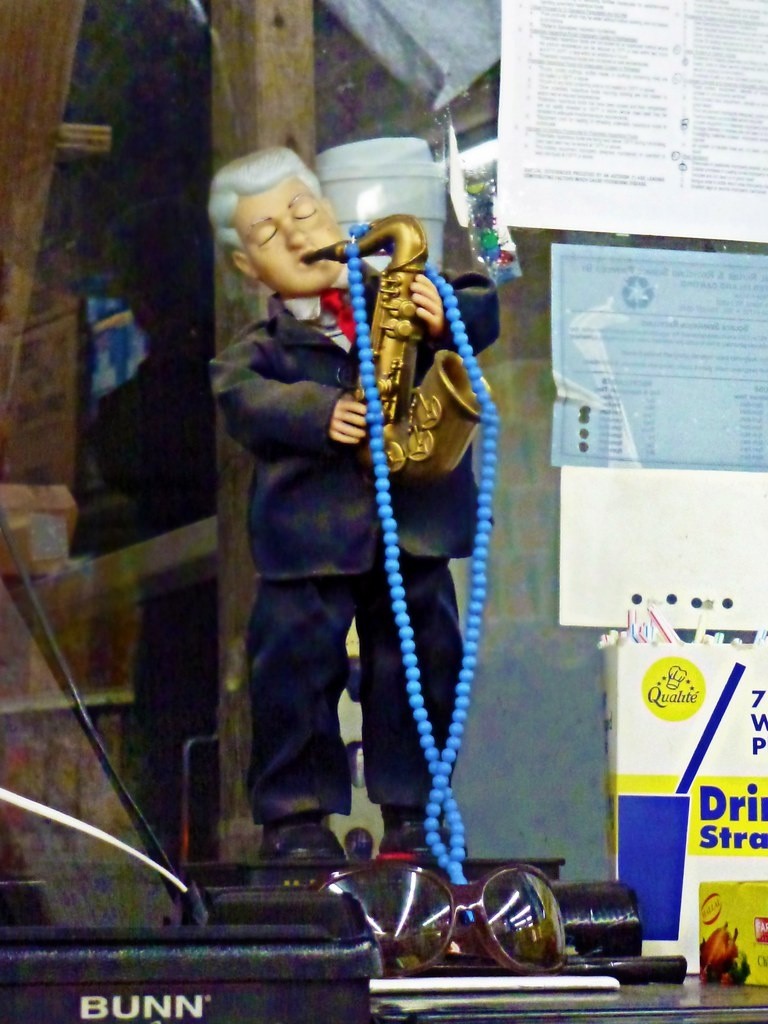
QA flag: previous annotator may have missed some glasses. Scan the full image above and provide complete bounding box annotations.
[315,866,566,979]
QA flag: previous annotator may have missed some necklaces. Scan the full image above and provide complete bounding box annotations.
[347,224,500,890]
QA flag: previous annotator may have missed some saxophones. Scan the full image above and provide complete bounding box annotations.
[304,214,493,476]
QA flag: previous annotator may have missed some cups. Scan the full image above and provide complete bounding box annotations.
[313,162,448,272]
[312,138,435,169]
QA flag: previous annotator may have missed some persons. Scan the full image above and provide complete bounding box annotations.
[202,149,499,858]
[91,204,220,833]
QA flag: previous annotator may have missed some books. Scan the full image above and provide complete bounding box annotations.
[183,866,685,995]
